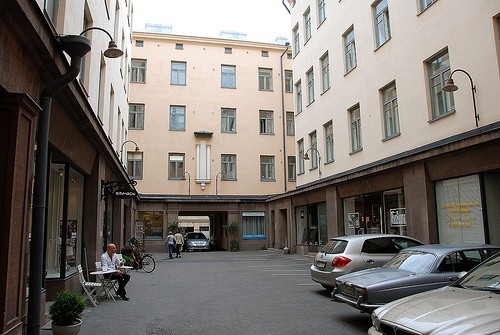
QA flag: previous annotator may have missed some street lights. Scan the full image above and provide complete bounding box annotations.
[121,141,140,162]
[303,146,322,173]
[180,172,191,197]
[80,26,125,57]
[443,70,479,125]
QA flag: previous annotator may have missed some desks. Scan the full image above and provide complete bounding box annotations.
[118,266,133,274]
[90,270,120,303]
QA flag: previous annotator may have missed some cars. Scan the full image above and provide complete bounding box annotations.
[331,243,500,314]
[369,252,500,334]
[184,231,211,250]
[312,233,423,290]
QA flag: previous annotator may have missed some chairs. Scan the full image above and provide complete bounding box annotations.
[77,254,124,307]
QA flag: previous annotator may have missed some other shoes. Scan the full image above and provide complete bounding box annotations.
[169,255,174,259]
[176,255,181,258]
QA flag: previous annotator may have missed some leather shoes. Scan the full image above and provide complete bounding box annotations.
[116,290,128,301]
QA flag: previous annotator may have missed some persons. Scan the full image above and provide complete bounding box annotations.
[100,243,131,301]
[174,227,184,258]
[165,231,176,259]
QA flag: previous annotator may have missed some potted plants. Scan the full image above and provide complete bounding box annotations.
[49,289,86,335]
[222,220,241,252]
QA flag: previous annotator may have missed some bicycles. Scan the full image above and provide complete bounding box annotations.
[132,246,156,273]
